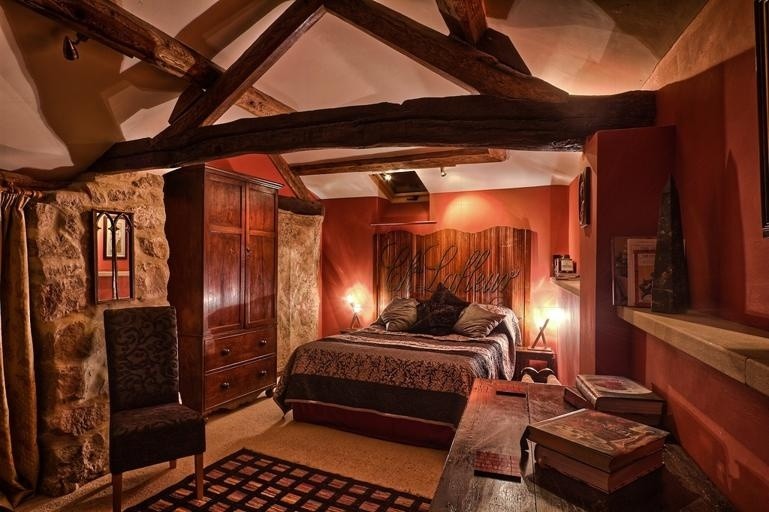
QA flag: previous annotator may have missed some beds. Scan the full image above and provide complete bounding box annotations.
[272,304,519,450]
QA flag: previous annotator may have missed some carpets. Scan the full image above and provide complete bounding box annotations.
[121,446,432,512]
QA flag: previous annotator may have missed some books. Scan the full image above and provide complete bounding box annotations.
[523,367,669,495]
[553,254,580,280]
[609,233,686,307]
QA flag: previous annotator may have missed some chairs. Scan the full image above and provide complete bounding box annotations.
[103,305,206,512]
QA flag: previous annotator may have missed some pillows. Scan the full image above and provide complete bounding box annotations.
[372,281,507,339]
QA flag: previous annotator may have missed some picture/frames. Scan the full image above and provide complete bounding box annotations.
[753,0,769,236]
[91,208,135,305]
[577,166,590,229]
[103,215,127,260]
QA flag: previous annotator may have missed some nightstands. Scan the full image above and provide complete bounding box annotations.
[339,328,361,334]
[516,345,556,382]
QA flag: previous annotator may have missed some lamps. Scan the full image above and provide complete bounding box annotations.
[440,167,446,176]
[62,33,90,61]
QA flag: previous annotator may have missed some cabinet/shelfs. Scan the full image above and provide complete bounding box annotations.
[161,164,285,422]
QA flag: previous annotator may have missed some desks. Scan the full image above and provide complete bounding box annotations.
[426,376,743,512]
